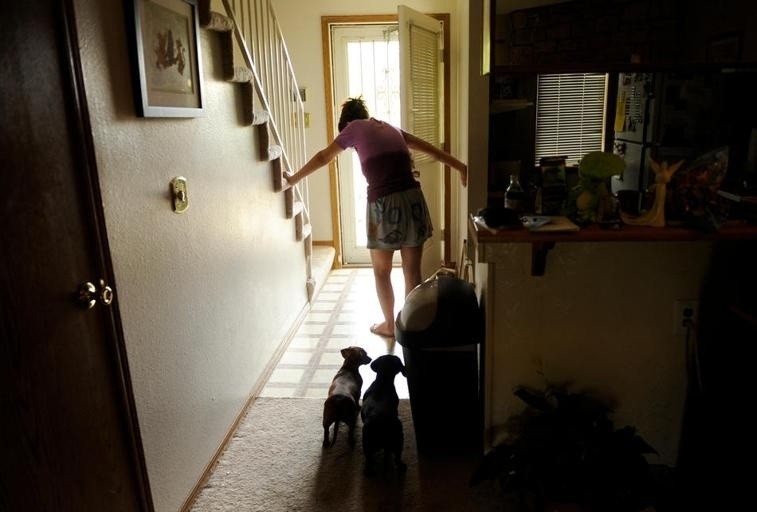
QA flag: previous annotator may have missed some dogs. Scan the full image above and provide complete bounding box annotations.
[320,346,371,449]
[358,353,408,479]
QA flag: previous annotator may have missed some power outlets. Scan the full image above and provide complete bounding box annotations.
[675,298,699,336]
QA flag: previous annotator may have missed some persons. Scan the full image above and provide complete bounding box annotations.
[281,92,468,340]
[653,160,671,225]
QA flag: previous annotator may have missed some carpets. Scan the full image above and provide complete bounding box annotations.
[187,396,498,512]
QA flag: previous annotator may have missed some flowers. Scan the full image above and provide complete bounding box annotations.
[472,373,661,508]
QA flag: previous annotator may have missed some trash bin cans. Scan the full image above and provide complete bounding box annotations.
[394,271,482,464]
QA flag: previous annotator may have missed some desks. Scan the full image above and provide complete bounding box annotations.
[470,215,706,472]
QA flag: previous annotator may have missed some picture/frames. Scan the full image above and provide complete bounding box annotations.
[129,0,207,120]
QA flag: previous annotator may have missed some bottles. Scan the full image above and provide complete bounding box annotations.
[503,173,526,214]
